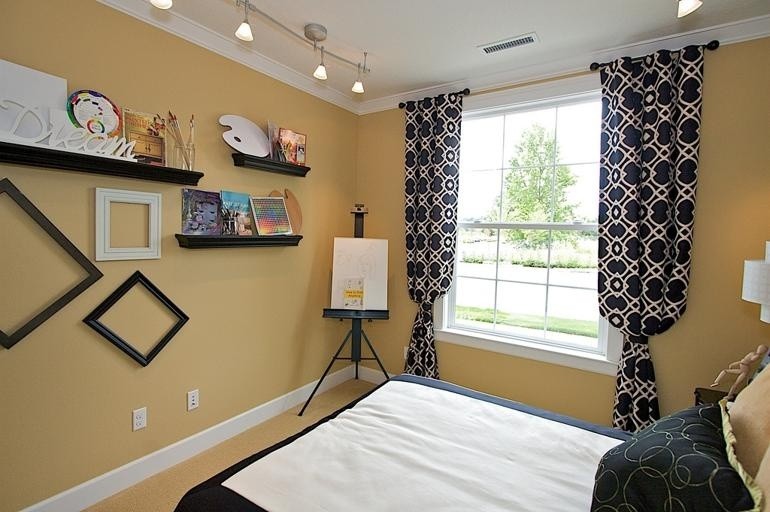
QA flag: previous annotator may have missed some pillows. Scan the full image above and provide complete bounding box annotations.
[590,364,770,512]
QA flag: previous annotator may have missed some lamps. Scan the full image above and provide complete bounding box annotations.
[741,241,770,324]
[235,0,370,94]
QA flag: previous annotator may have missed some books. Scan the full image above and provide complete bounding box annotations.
[277,128,307,167]
[120,107,168,168]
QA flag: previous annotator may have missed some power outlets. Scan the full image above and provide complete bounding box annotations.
[133,407,147,431]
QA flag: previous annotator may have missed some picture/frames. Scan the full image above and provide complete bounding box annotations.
[82,270,189,367]
[96,188,162,262]
[0,177,104,350]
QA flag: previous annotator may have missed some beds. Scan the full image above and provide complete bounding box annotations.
[174,372,637,512]
